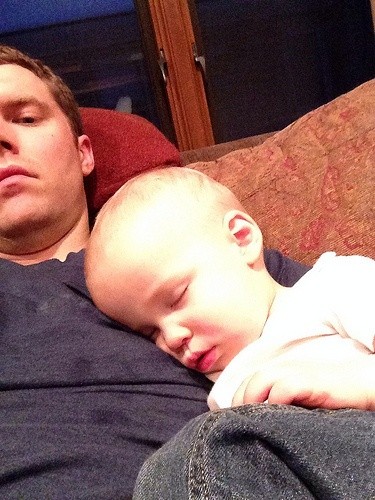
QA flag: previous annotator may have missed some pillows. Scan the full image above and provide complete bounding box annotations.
[184,78,375,266]
[77,108,184,215]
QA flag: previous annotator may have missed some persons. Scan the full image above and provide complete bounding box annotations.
[0,44,375,500]
[84,166,375,410]
